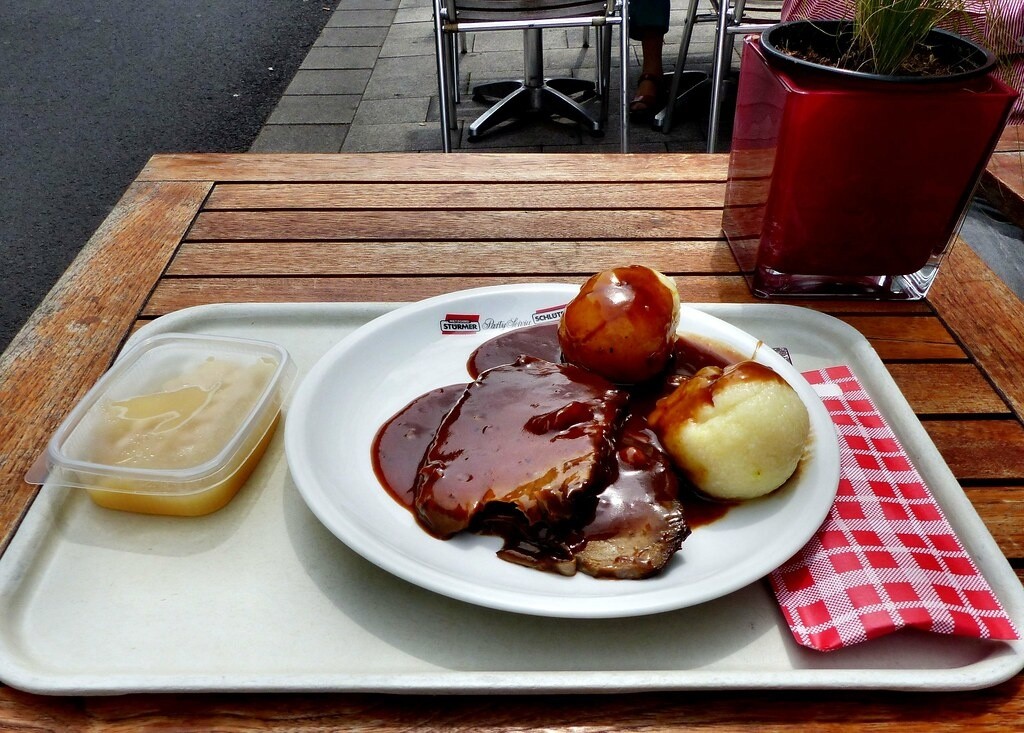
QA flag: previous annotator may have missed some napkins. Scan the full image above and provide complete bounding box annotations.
[767,366,1023,651]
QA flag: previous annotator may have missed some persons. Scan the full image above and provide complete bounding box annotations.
[628,0,669,114]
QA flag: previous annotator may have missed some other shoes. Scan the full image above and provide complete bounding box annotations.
[629,73,664,115]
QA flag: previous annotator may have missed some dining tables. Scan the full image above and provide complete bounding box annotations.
[0,152,1024,733]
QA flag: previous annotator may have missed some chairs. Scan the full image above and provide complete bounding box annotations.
[432,0,782,152]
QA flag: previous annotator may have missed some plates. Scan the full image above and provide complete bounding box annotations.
[285,283,840,619]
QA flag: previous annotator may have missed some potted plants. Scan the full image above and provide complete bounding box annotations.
[722,0,1024,304]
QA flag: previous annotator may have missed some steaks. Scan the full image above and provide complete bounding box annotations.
[417,361,690,578]
